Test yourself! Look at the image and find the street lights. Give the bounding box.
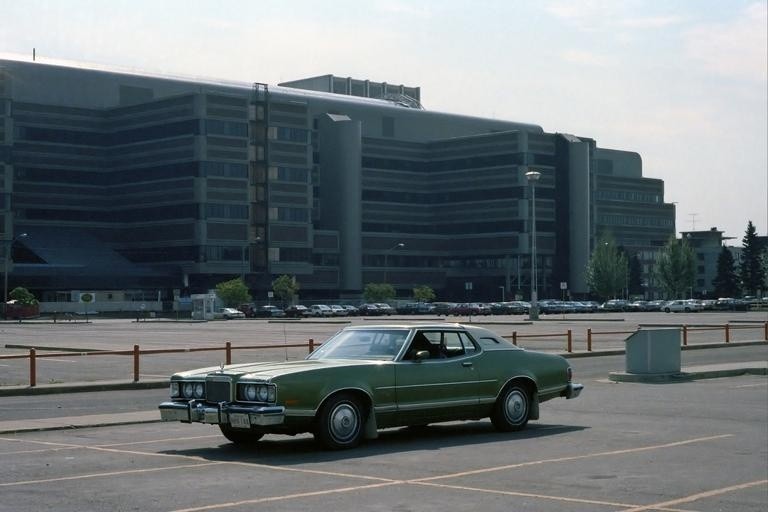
[241,236,262,281]
[522,168,545,320]
[384,243,404,282]
[4,231,29,319]
[498,286,505,302]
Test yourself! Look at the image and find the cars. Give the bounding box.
[156,319,586,444]
